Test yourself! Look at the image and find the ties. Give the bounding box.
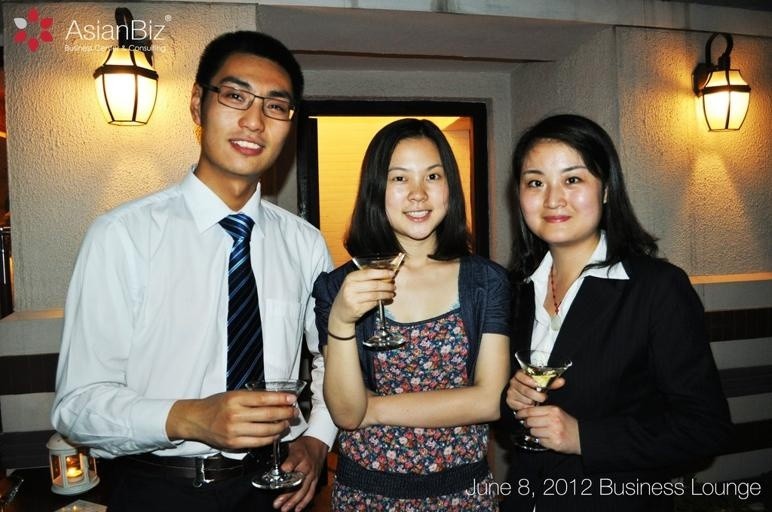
[218,215,269,394]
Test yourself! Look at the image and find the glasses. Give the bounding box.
[204,82,300,122]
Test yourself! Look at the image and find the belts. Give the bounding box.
[128,453,250,485]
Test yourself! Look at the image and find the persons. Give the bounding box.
[52,30,338,511]
[488,114,734,510]
[313,119,512,511]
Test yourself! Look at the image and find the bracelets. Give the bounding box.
[327,326,356,342]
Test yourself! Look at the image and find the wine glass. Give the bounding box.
[352,253,407,348]
[246,378,306,490]
[514,350,573,451]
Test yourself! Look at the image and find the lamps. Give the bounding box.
[690,30,751,133]
[91,7,159,128]
